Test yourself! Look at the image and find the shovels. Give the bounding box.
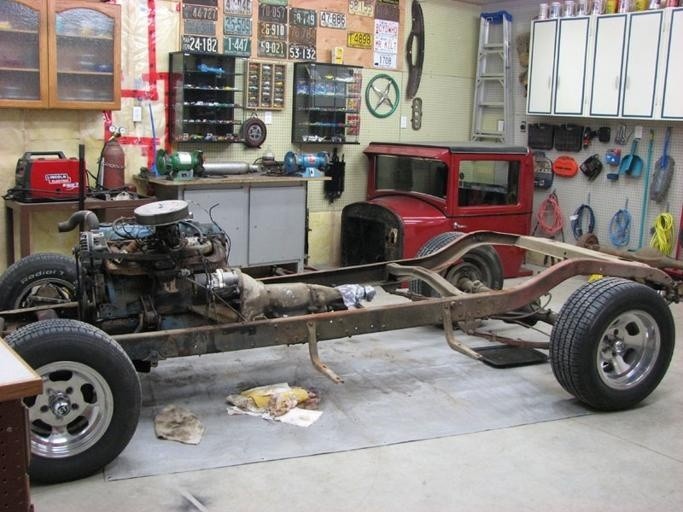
[619,139,643,177]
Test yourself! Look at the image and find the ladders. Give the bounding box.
[470,10,514,145]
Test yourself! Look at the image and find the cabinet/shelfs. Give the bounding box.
[168,50,250,144]
[525,7,682,122]
[292,61,364,144]
[0,0,122,110]
[4,193,157,268]
[243,60,288,111]
[0,333,44,512]
[155,181,308,273]
